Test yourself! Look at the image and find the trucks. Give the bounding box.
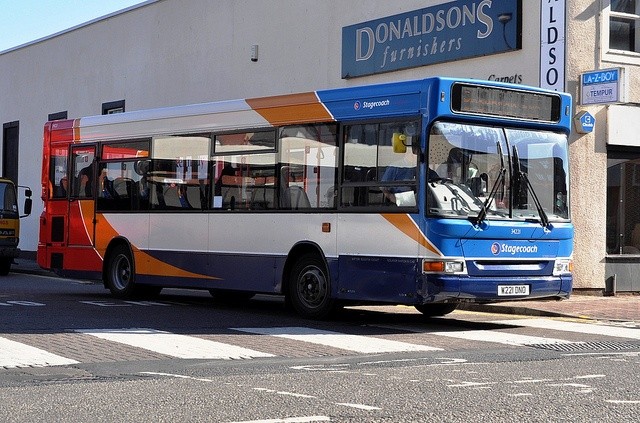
[0,175,34,276]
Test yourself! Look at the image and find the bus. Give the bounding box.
[36,76,576,323]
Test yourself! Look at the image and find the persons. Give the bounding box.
[76,156,100,198]
[434,147,481,197]
[380,141,428,204]
